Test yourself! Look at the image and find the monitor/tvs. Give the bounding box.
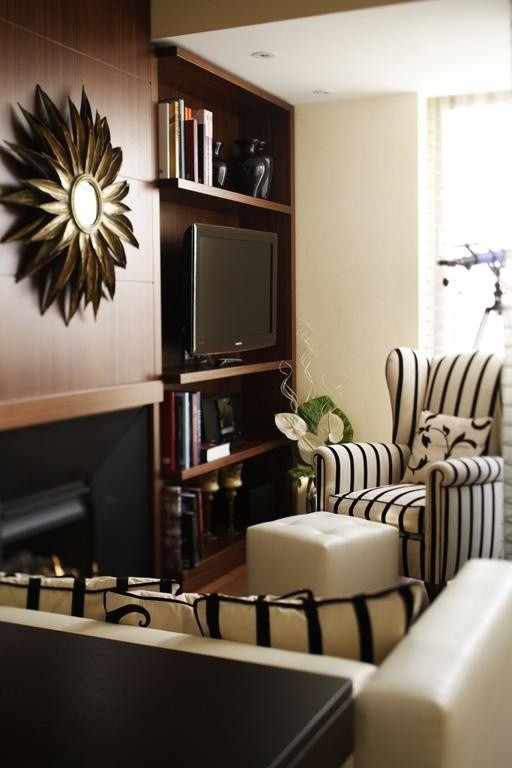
[182,221,278,356]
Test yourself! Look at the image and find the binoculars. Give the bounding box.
[437,245,512,286]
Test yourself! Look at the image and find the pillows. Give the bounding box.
[402,408,494,488]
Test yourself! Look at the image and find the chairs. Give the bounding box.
[308,346,510,597]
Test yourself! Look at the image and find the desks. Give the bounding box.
[0,622,356,767]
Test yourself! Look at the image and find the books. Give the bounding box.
[167,390,243,470]
[160,98,214,188]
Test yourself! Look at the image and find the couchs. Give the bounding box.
[0,556,512,767]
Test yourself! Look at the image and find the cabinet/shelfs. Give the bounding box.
[151,40,300,596]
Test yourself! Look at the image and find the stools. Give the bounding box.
[245,510,399,598]
